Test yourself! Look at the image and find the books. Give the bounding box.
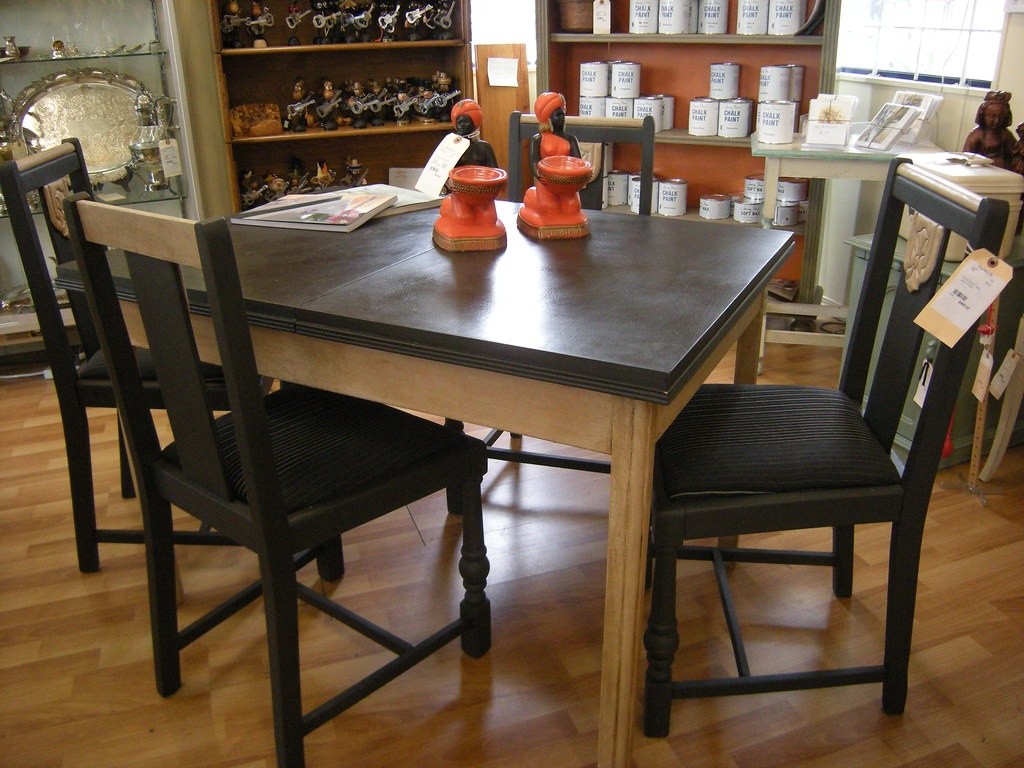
[231,182,445,234]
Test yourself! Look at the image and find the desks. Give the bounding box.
[52,199,794,768]
[751,129,952,376]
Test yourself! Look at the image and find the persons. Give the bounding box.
[220,0,457,49]
[964,89,1024,235]
[281,72,453,131]
[239,157,368,210]
[524,91,594,216]
[438,99,508,227]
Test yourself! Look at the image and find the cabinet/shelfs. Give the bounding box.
[535,1,842,331]
[206,0,470,218]
[0,1,210,362]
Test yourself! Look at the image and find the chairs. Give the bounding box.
[62,201,495,768]
[643,155,1009,740]
[1,137,276,576]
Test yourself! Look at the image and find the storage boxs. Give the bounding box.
[893,151,1024,262]
[846,235,1024,470]
